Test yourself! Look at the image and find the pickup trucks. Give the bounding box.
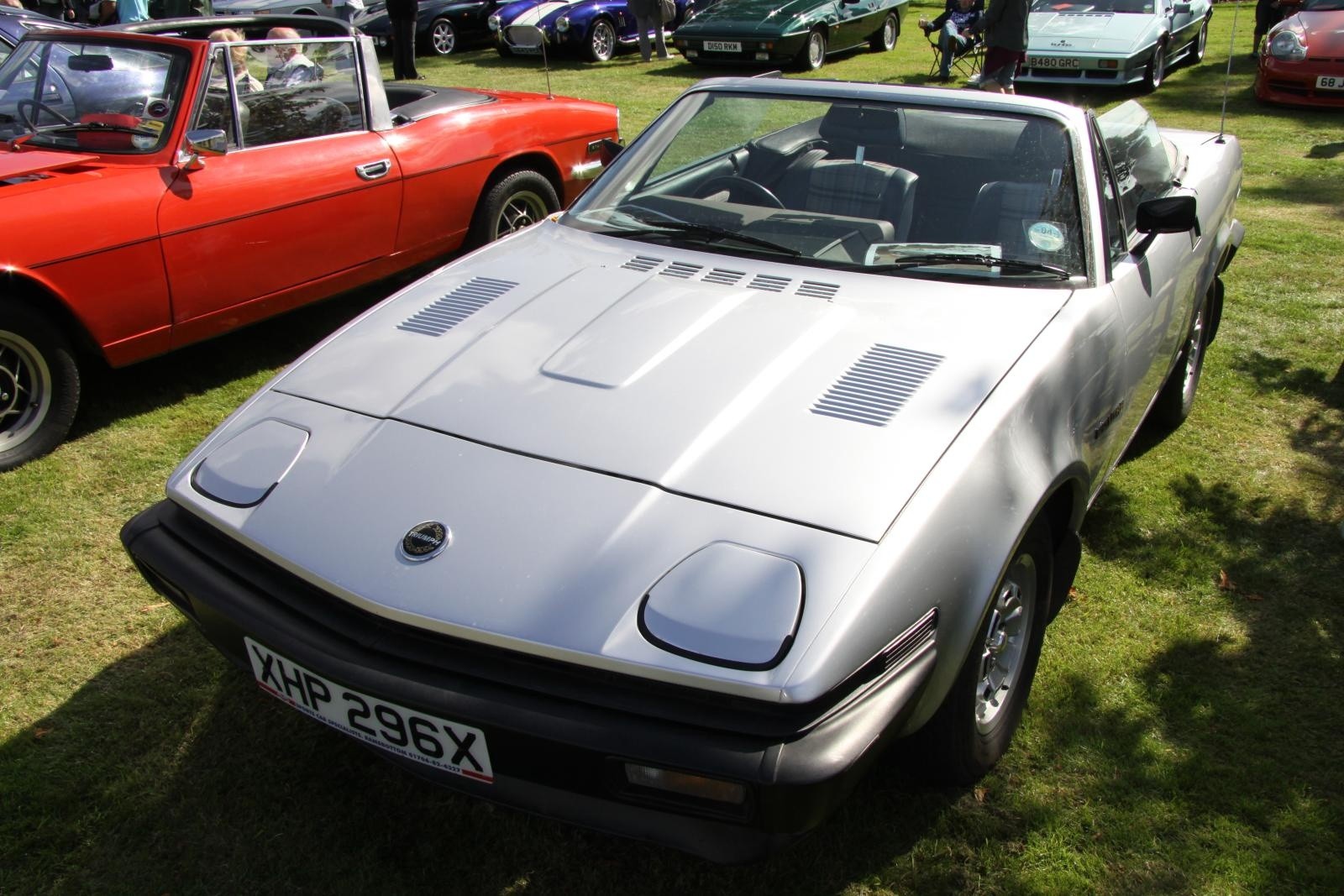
[117,68,1247,868]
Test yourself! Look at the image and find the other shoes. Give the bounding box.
[645,57,651,62]
[939,76,949,84]
[417,74,427,81]
[946,35,958,51]
[659,53,674,60]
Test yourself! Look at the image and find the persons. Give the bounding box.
[626,0,721,62]
[207,28,264,95]
[316,0,336,36]
[1249,0,1288,61]
[0,0,215,27]
[918,0,1034,95]
[385,0,428,81]
[263,27,324,91]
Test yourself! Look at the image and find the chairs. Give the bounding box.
[776,102,920,243]
[924,0,986,79]
[970,120,1085,265]
[124,87,352,148]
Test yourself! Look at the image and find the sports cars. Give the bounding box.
[0,10,628,474]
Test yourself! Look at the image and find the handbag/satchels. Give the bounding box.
[660,0,676,23]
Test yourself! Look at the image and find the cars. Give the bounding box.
[194,0,696,65]
[0,6,172,145]
[1251,0,1344,112]
[669,0,913,72]
[1013,1,1216,95]
